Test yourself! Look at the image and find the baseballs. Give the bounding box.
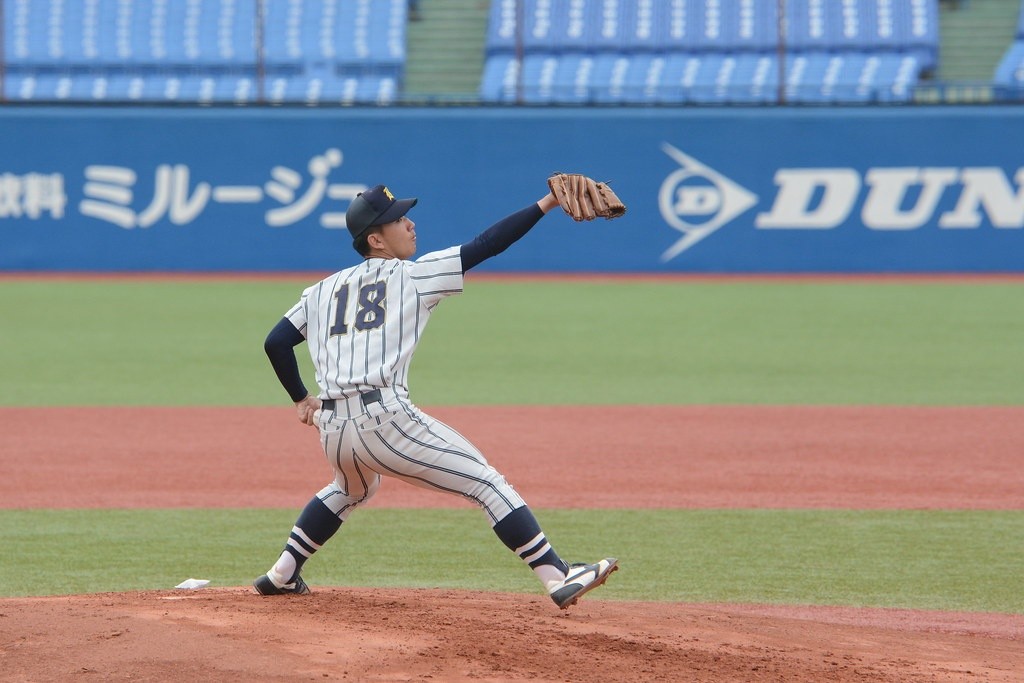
[313,409,320,426]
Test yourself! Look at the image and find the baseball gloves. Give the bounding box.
[547,172,626,222]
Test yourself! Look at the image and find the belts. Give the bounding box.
[322,389,381,410]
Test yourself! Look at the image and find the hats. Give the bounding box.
[346,185,417,240]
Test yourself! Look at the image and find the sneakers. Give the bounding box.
[550,558,619,610]
[253,567,311,596]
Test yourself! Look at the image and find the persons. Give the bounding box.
[253,171,627,610]
